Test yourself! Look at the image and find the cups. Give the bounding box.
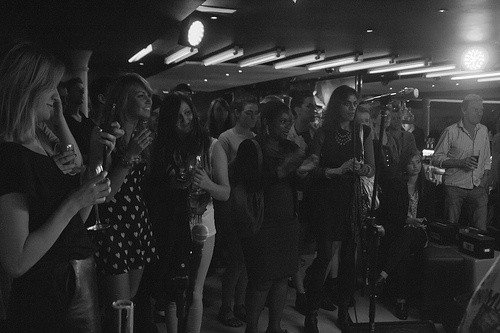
[53,143,75,175]
[470,150,480,169]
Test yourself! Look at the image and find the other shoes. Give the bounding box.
[319,289,337,312]
[233,304,249,322]
[217,311,242,327]
[396,302,408,320]
[349,296,354,308]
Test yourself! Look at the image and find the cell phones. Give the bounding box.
[102,103,118,132]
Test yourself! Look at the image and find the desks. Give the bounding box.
[458,248,500,333]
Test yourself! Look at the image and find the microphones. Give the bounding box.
[360,87,420,104]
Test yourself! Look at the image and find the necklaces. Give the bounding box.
[335,138,352,144]
[336,128,350,138]
[338,130,352,141]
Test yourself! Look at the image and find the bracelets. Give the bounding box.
[120,155,133,169]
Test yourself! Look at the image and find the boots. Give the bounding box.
[304,311,319,333]
[339,305,356,333]
[295,293,318,317]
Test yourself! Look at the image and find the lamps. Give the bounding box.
[126,19,500,82]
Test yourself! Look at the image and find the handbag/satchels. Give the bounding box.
[358,124,380,210]
[231,138,264,224]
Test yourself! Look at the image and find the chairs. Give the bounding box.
[364,225,434,333]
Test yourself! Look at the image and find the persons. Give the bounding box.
[0,40,500,333]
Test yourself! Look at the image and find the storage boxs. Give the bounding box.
[456,229,496,259]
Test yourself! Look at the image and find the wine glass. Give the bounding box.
[80,161,111,230]
[187,154,207,196]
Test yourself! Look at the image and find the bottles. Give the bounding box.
[423,136,437,150]
